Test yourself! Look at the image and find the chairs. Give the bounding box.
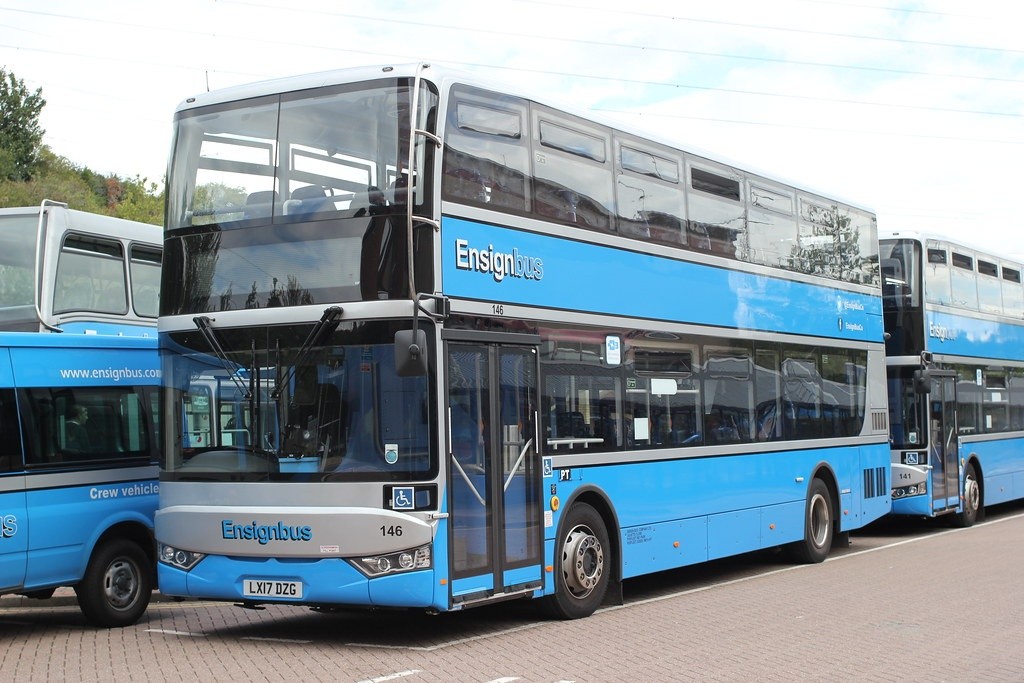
[289,184,337,216]
[135,283,158,316]
[349,187,386,210]
[244,191,281,220]
[386,176,417,205]
[62,274,94,310]
[96,278,125,312]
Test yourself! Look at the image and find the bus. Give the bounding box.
[0,199,279,629]
[156,58,893,623]
[781,230,1024,529]
